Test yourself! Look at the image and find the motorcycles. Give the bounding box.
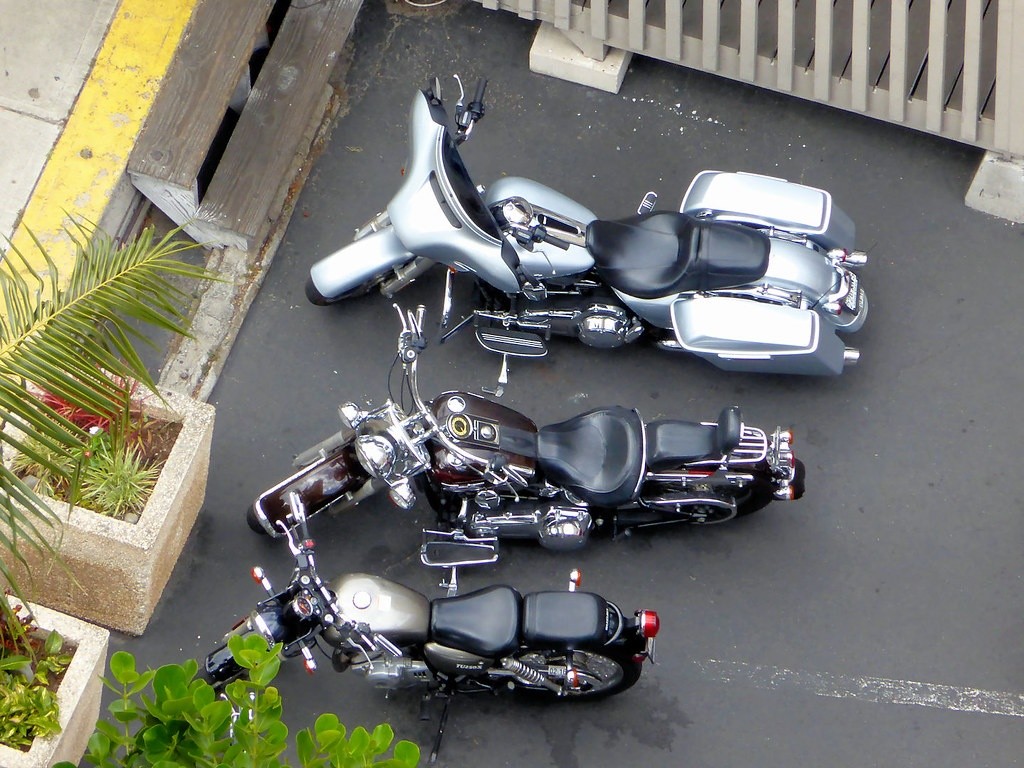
[304,73,869,397]
[242,302,805,596]
[187,491,662,765]
